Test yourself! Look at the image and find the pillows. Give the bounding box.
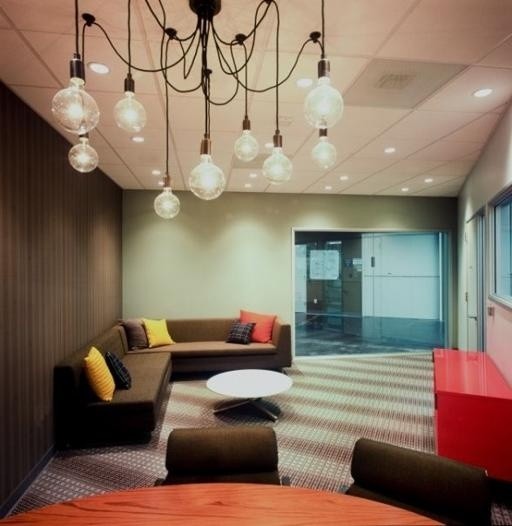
[225,320,255,345]
[105,351,131,389]
[141,318,175,348]
[84,346,116,402]
[239,310,277,343]
[117,318,149,350]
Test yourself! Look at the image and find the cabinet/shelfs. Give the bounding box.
[432,348,512,480]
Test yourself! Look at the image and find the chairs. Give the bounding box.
[154,426,289,486]
[345,438,490,525]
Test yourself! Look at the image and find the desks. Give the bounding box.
[0,482,446,526]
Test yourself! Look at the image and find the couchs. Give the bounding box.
[116,317,291,373]
[52,328,172,432]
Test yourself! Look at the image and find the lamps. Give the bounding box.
[51,0,345,220]
